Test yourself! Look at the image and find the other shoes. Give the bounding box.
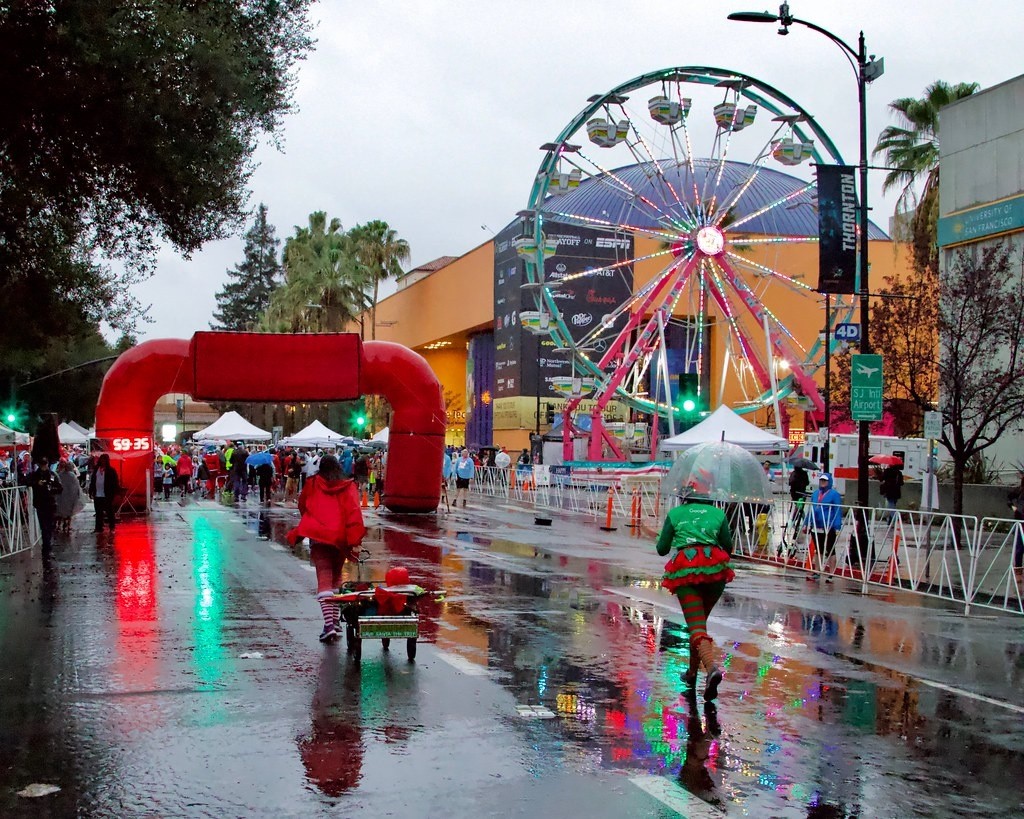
[111,528,115,535]
[260,501,265,503]
[320,623,336,642]
[158,497,162,501]
[680,671,696,689]
[826,577,833,584]
[332,620,344,634]
[164,497,167,501]
[181,491,187,498]
[463,500,466,507]
[241,496,247,501]
[268,500,272,507]
[809,574,821,579]
[154,497,158,501]
[452,501,457,506]
[91,530,104,535]
[703,670,722,702]
[49,550,56,558]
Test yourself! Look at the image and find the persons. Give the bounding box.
[31,457,63,564]
[153,438,529,506]
[714,501,771,537]
[656,467,734,700]
[284,455,366,641]
[874,465,904,528]
[803,473,843,581]
[0,442,95,493]
[88,454,118,533]
[763,460,774,480]
[788,465,809,527]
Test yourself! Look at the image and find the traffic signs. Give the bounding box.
[850,353,888,422]
[7,411,20,428]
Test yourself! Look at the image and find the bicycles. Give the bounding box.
[775,491,815,559]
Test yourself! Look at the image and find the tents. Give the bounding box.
[0,420,31,483]
[67,420,91,435]
[660,403,788,535]
[542,417,592,465]
[56,419,89,454]
[362,426,390,449]
[192,409,273,456]
[279,416,363,446]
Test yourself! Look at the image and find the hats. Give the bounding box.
[819,475,829,481]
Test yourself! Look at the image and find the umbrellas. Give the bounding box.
[660,429,775,508]
[495,452,511,469]
[868,454,904,466]
[778,456,820,470]
[245,452,275,465]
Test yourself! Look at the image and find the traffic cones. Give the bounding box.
[360,490,371,509]
[371,491,381,509]
[521,474,531,490]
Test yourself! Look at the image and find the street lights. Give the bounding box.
[728,2,883,565]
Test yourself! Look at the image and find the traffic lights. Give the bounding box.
[18,402,29,425]
[356,412,368,429]
